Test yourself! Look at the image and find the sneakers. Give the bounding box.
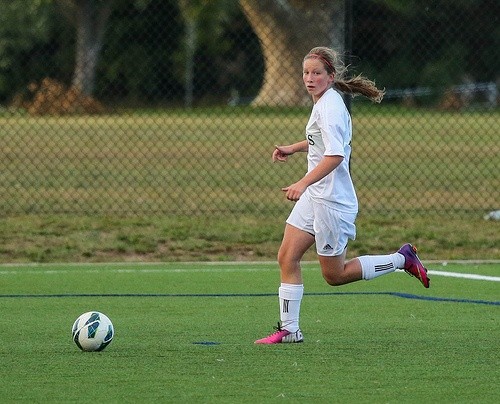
[397,243,431,289]
[254,322,304,344]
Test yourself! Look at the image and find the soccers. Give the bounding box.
[71,311,115,352]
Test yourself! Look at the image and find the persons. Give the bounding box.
[254,46,430,344]
